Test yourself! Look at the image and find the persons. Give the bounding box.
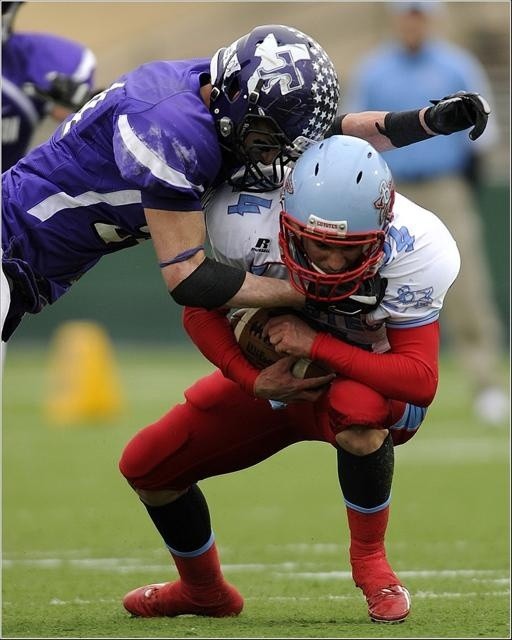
[0,0,100,171]
[1,25,491,354]
[339,4,511,436]
[115,136,462,620]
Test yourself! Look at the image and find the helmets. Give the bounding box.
[207,21,342,197]
[274,133,398,305]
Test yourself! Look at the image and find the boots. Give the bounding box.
[122,528,246,620]
[343,493,413,626]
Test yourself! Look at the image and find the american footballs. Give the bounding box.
[230,308,341,393]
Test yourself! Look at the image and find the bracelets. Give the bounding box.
[383,109,430,148]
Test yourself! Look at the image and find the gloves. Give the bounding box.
[300,267,388,319]
[418,87,491,142]
